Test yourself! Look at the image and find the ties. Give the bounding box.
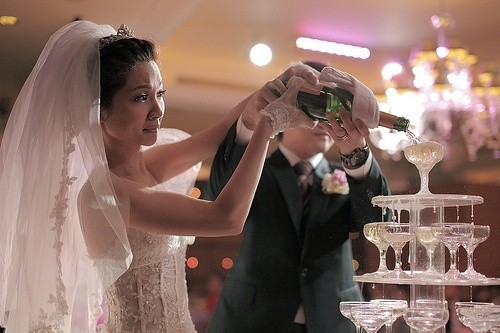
[294,161,315,232]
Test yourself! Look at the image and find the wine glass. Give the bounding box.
[405,142,445,194]
[339,299,500,333]
[363,221,490,279]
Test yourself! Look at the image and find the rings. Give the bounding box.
[338,132,349,142]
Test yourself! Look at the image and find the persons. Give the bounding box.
[187,266,500,333]
[77,24,319,333]
[205,61,391,333]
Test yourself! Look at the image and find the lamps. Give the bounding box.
[368,14,500,167]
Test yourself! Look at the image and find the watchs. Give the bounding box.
[339,141,370,170]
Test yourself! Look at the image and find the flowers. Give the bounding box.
[321,169,351,195]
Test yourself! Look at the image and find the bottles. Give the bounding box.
[281,77,409,134]
